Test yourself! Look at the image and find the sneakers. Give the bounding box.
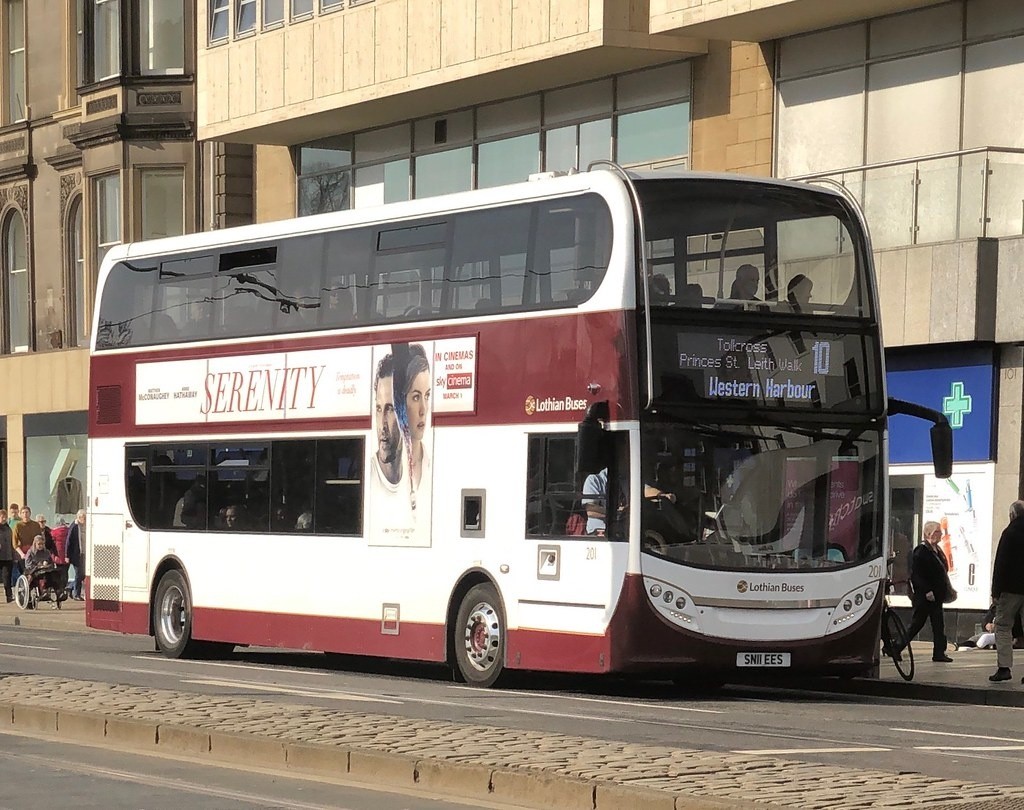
[988,668,1012,681]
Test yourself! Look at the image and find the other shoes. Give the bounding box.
[932,657,953,662]
[57,592,69,603]
[947,641,958,651]
[7,595,15,602]
[66,590,73,599]
[891,643,903,661]
[37,591,49,602]
[74,595,85,601]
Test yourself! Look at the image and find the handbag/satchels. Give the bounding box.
[943,572,957,603]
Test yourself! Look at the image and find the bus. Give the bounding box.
[82,159,954,688]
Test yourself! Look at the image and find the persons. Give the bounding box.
[224,505,245,531]
[788,274,814,314]
[717,264,769,310]
[1,504,86,601]
[988,500,1024,685]
[889,521,958,662]
[373,346,431,498]
[330,281,354,325]
[582,435,676,538]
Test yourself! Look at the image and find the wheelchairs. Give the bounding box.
[15,555,70,610]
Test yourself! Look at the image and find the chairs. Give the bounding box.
[159,313,178,338]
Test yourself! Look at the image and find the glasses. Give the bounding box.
[38,520,46,524]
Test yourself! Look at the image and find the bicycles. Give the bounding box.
[881,551,914,681]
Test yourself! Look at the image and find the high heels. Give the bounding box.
[882,645,891,656]
